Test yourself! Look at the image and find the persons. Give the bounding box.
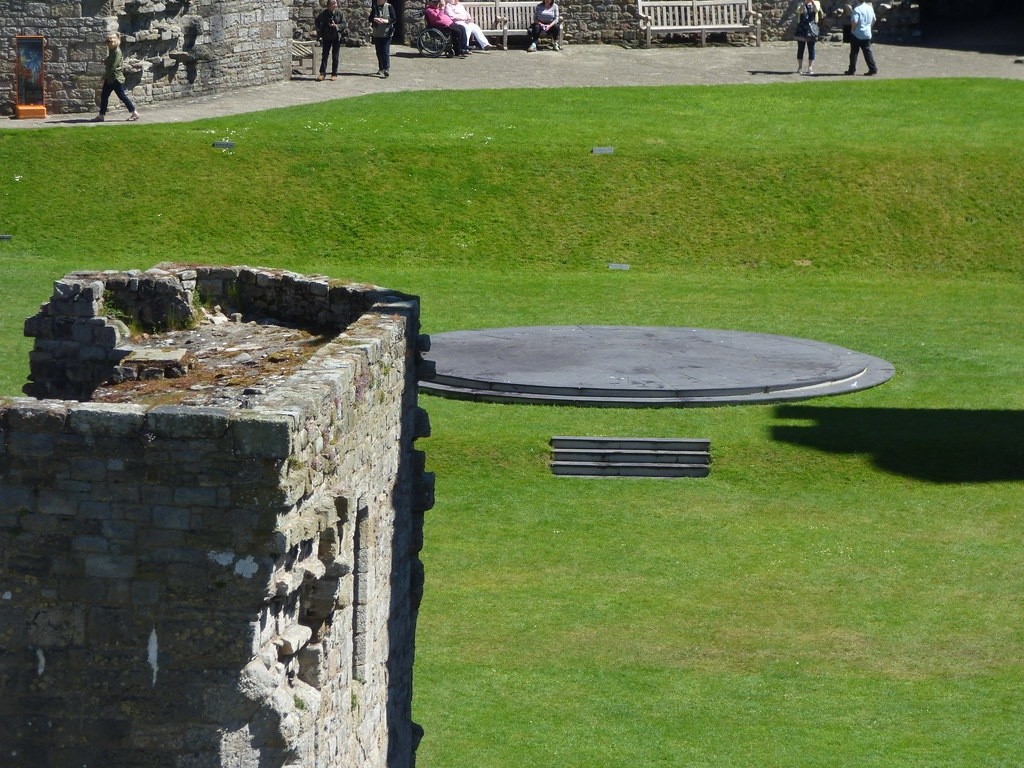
[444,0,497,51]
[91,34,140,122]
[368,0,396,77]
[425,0,476,55]
[794,0,825,74]
[844,0,877,75]
[528,0,560,51]
[315,0,346,81]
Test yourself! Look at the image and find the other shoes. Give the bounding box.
[844,71,856,75]
[552,43,560,51]
[527,46,538,52]
[467,46,475,51]
[126,114,140,121]
[331,75,337,80]
[377,70,383,75]
[864,70,877,76]
[384,70,389,77]
[317,75,325,81]
[91,116,104,122]
[797,70,803,74]
[484,44,497,51]
[808,69,813,74]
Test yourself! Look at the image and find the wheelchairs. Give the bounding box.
[415,8,459,60]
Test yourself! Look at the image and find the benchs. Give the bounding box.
[292,40,321,74]
[461,2,564,51]
[637,0,763,49]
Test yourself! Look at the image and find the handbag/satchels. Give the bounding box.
[805,4,820,38]
